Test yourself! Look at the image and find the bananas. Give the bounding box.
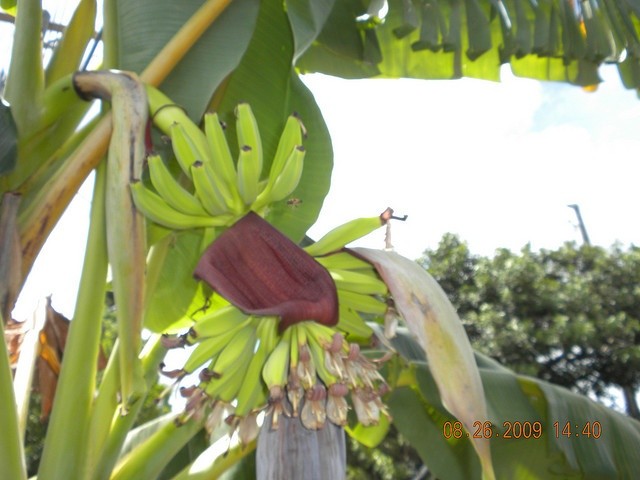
[129,101,304,231]
[182,216,388,419]
